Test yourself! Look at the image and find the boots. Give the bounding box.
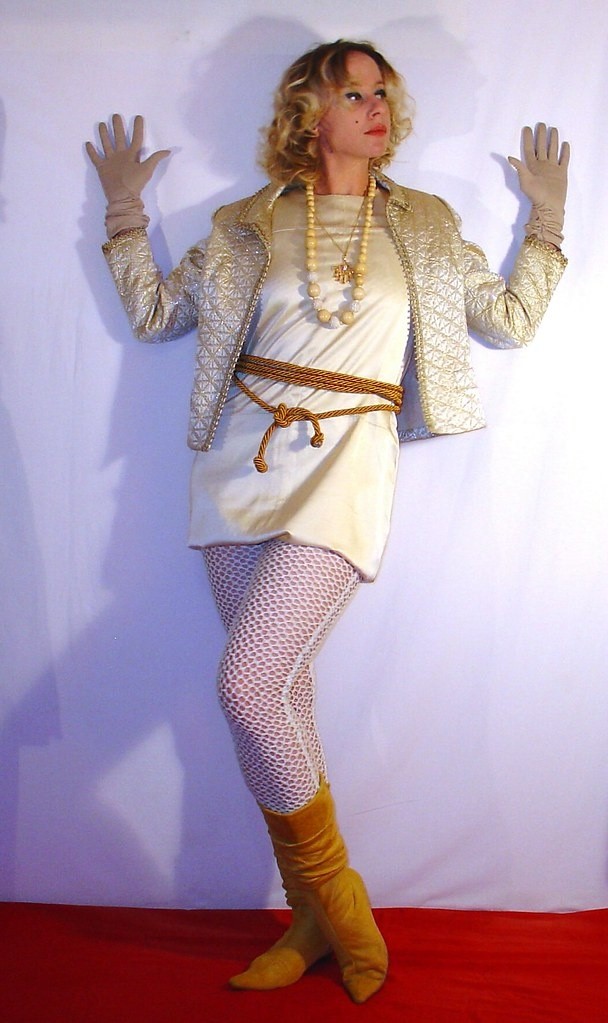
[255,770,390,1005]
[230,828,336,991]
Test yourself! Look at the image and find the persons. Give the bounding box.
[85,40,571,1004]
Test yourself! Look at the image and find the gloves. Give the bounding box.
[86,112,171,241]
[507,122,571,251]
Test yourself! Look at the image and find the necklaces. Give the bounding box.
[301,173,377,329]
